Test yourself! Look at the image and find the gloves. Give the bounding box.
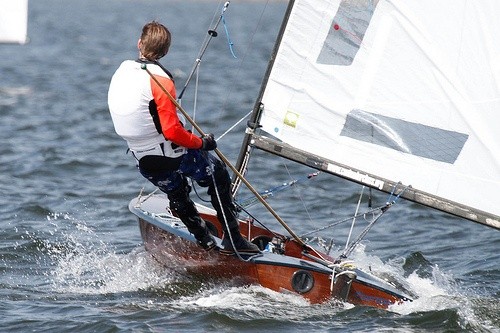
[200,132,218,152]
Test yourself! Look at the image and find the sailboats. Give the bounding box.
[127,0,500,316]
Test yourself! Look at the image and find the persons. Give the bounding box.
[107,20,265,256]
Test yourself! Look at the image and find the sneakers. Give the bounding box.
[195,235,218,258]
[218,227,262,258]
